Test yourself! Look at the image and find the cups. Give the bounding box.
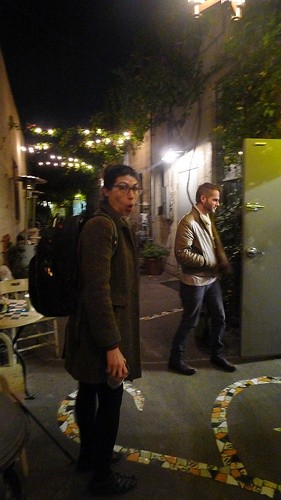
[107,364,130,389]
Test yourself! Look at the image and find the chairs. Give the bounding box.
[0,278,62,370]
[0,332,32,500]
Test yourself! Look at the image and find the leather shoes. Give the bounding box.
[210,358,239,372]
[77,450,139,497]
[168,361,198,375]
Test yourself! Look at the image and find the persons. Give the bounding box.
[167,182,238,374]
[64,163,143,495]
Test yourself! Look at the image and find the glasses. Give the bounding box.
[112,182,144,196]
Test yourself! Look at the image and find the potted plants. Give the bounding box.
[142,243,167,275]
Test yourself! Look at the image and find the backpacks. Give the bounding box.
[27,213,119,318]
